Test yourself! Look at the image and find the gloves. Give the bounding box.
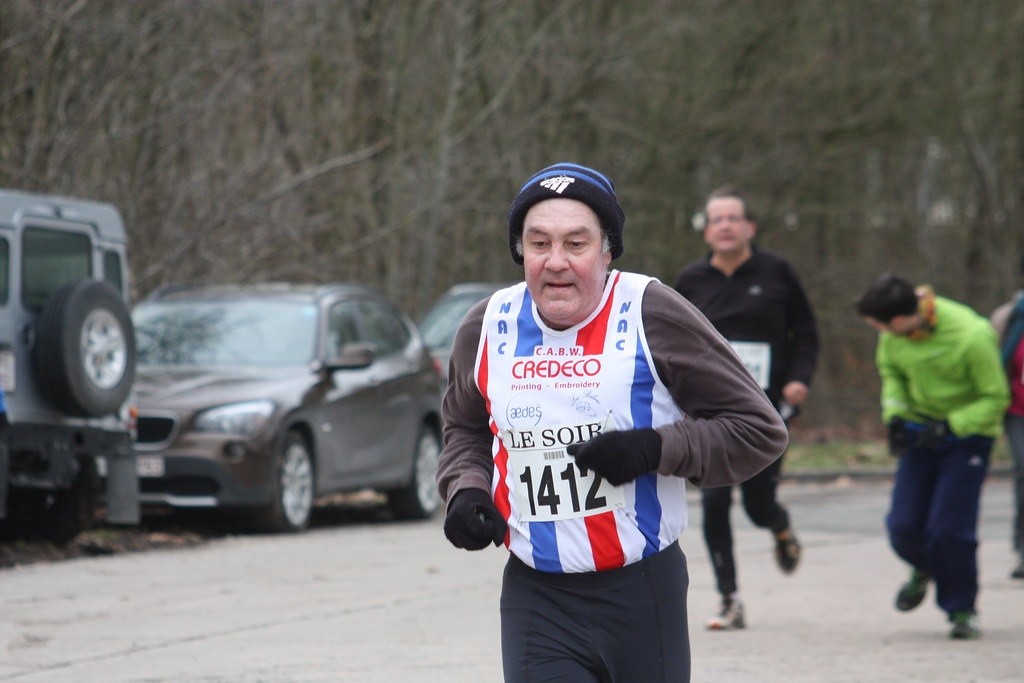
[567,428,662,487]
[444,487,508,553]
[917,422,957,452]
[888,418,909,455]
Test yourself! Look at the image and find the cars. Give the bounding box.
[97,278,445,535]
[419,279,523,383]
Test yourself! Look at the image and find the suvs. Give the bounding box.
[0,186,142,547]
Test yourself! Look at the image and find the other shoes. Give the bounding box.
[774,525,799,572]
[708,597,743,631]
[950,611,974,636]
[896,570,931,612]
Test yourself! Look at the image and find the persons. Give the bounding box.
[854,273,1009,637]
[673,183,818,630]
[989,289,1024,579]
[433,162,788,683]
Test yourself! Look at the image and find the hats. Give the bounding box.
[507,163,625,264]
